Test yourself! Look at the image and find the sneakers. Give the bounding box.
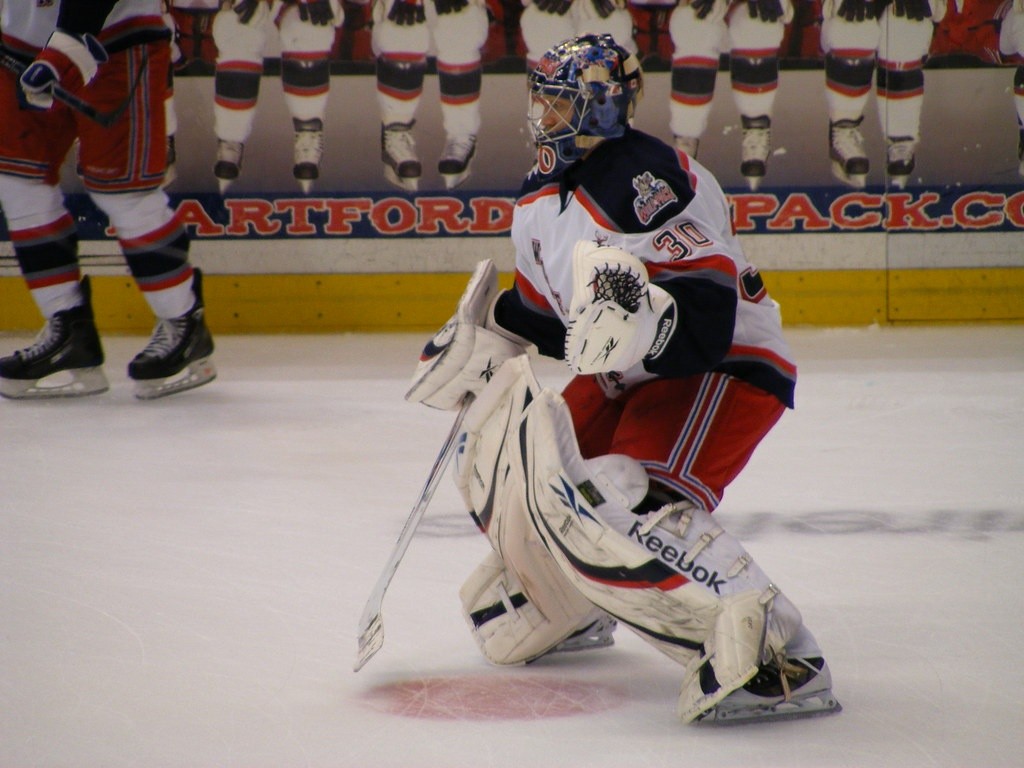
[742,113,772,189]
[290,118,326,193]
[127,266,222,402]
[825,120,873,186]
[887,137,918,187]
[0,273,110,399]
[438,132,481,190]
[211,139,247,192]
[701,632,845,722]
[379,120,426,190]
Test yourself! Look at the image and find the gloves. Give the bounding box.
[385,2,468,28]
[537,1,627,21]
[836,1,933,24]
[691,0,789,25]
[229,1,340,28]
[20,33,107,113]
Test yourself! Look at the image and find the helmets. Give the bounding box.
[529,33,643,139]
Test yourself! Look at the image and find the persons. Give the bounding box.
[0,0,213,379]
[405,33,833,724]
[74,0,1024,180]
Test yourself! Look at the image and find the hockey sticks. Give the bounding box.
[354,393,473,672]
[0,43,153,128]
